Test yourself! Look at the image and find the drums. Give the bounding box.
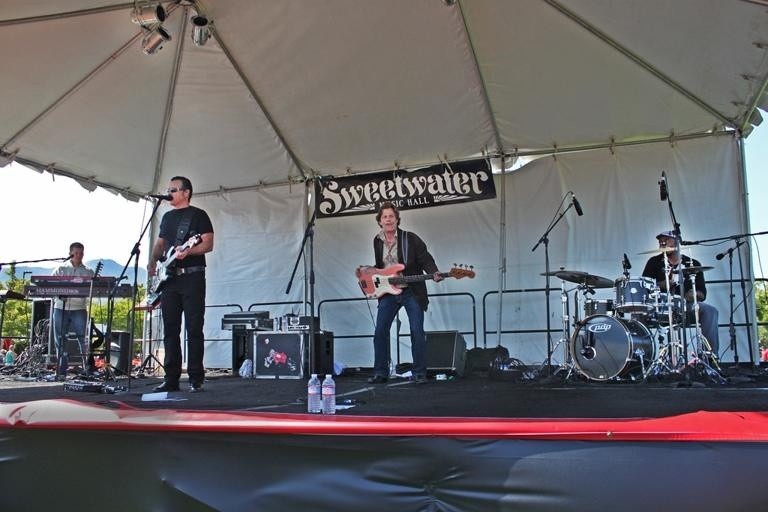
[585,299,615,317]
[615,276,659,316]
[570,314,656,384]
[648,293,688,326]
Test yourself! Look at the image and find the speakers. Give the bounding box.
[424,330,466,377]
[64,255,74,263]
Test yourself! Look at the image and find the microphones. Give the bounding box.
[659,177,667,201]
[716,241,746,261]
[572,192,583,216]
[623,252,631,270]
[150,194,172,201]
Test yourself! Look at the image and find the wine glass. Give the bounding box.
[290,316,300,330]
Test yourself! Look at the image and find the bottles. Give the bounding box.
[322,374,336,414]
[307,374,320,413]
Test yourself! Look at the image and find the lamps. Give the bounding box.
[130,0,216,56]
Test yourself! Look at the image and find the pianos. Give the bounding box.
[22,276,135,298]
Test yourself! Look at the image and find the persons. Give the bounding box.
[354,204,444,384]
[642,231,722,375]
[44,242,95,381]
[147,177,214,393]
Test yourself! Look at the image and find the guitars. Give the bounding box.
[149,234,203,292]
[357,263,476,300]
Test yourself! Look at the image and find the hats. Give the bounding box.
[656,231,676,237]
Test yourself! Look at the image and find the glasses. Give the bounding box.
[165,187,184,193]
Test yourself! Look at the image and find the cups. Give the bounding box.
[282,314,288,331]
[273,315,279,330]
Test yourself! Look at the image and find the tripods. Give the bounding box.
[646,254,725,384]
[538,280,571,378]
[564,294,585,383]
[91,300,136,383]
[133,310,164,375]
[684,274,727,377]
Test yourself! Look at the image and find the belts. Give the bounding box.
[176,265,205,276]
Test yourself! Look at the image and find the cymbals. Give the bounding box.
[539,272,588,277]
[556,271,615,288]
[672,266,714,274]
[637,245,693,256]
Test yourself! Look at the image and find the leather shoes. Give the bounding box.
[153,381,181,393]
[368,374,387,383]
[413,375,427,384]
[188,381,205,394]
